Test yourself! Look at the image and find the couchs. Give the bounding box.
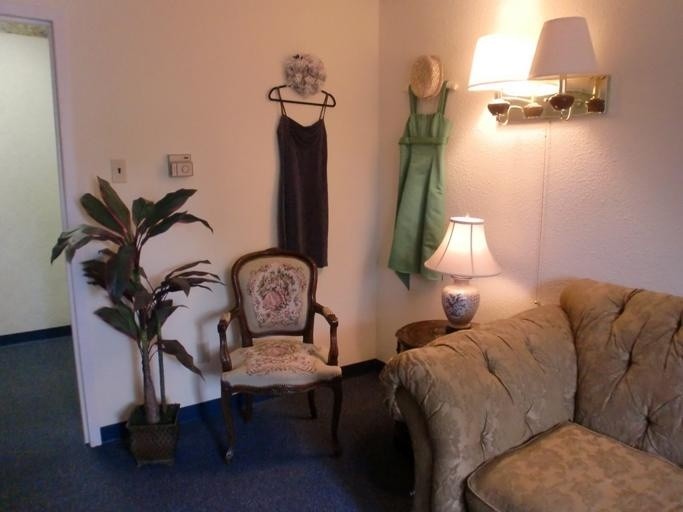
[375,281,683,512]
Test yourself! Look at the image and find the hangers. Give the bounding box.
[268,83,336,108]
[403,78,458,96]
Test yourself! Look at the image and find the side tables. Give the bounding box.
[394,317,482,353]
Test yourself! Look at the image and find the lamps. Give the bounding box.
[466,18,609,124]
[425,215,499,328]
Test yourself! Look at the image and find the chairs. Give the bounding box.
[214,246,344,464]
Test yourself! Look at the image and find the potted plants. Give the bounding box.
[49,175,226,466]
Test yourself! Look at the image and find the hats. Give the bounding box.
[409,56,443,98]
